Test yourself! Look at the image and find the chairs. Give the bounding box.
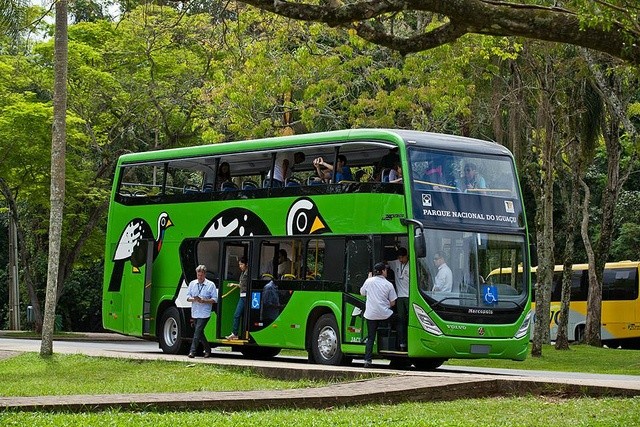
[380,167,392,183]
[118,173,332,197]
[259,269,324,281]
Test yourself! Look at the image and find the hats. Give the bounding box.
[374,262,390,273]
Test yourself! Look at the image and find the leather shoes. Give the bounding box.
[188,352,194,358]
[205,350,211,358]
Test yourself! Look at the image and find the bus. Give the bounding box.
[102,128,538,369]
[484,259,640,349]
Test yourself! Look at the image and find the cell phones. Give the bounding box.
[193,296,201,299]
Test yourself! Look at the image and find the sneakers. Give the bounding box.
[401,347,407,352]
[364,361,372,368]
[225,332,239,340]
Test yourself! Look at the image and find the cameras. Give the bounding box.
[313,158,320,165]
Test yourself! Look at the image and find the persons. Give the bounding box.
[389,162,403,183]
[215,162,232,191]
[458,162,485,193]
[187,265,218,358]
[360,262,399,368]
[423,159,445,191]
[385,247,409,348]
[381,146,403,173]
[225,257,249,340]
[267,152,290,183]
[278,249,291,278]
[432,250,453,292]
[313,154,352,185]
[262,276,280,320]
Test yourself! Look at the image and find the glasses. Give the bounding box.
[464,168,471,172]
[433,258,440,260]
